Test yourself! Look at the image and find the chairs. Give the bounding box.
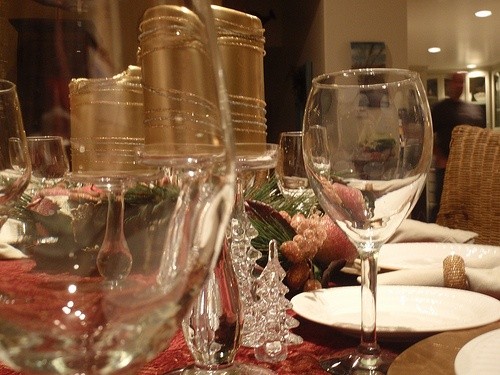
[434,126,499,244]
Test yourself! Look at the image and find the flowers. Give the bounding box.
[1,174,382,293]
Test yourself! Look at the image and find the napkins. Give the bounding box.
[356,218,500,300]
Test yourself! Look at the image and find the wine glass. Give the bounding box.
[302,68,434,375]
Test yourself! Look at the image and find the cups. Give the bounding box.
[275,132,319,211]
[1,0,236,375]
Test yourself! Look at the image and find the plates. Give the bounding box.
[453,328,500,375]
[375,242,500,270]
[357,266,500,298]
[289,284,500,341]
